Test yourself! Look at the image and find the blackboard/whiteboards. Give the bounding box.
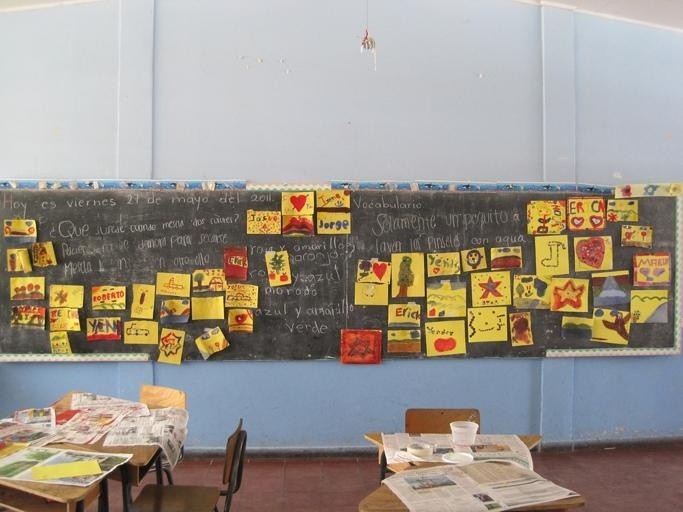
[0,180,683,363]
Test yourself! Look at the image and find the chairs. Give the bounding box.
[406,408,480,435]
[139,386,186,485]
[130,418,247,512]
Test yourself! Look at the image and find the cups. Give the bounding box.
[451,421,479,445]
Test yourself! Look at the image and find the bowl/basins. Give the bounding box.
[410,443,433,456]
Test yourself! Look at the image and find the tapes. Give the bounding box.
[407,441,437,457]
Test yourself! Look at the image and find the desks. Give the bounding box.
[359,433,585,512]
[0,441,113,512]
[40,391,173,512]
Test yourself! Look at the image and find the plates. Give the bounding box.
[444,452,473,462]
